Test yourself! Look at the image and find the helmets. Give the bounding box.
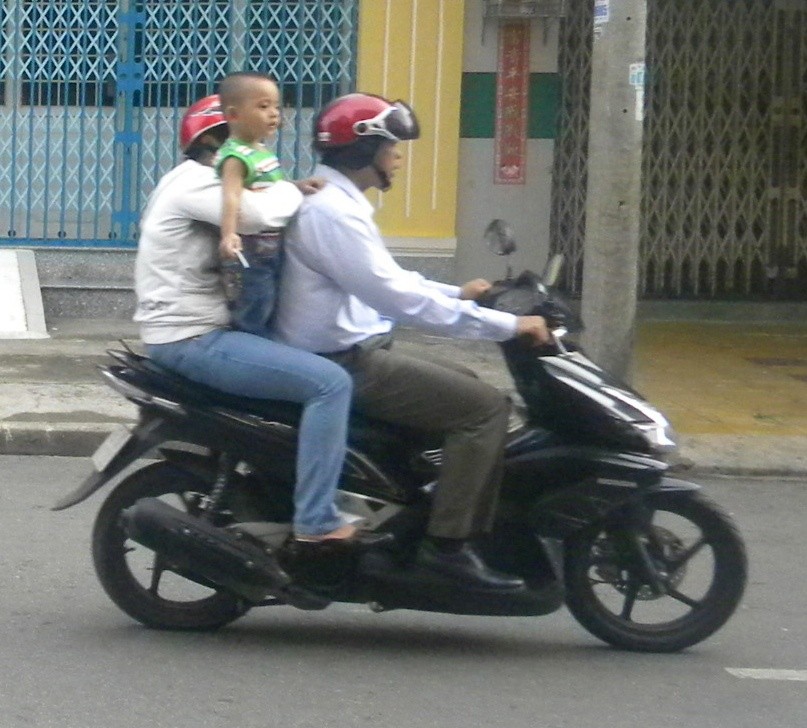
[312,91,420,150]
[179,94,227,154]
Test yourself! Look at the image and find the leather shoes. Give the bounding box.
[415,539,527,593]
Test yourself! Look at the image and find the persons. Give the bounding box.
[273,92,547,597]
[213,71,327,338]
[131,92,371,543]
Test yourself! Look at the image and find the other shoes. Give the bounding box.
[293,527,395,552]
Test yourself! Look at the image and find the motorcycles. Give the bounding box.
[51,216,751,653]
